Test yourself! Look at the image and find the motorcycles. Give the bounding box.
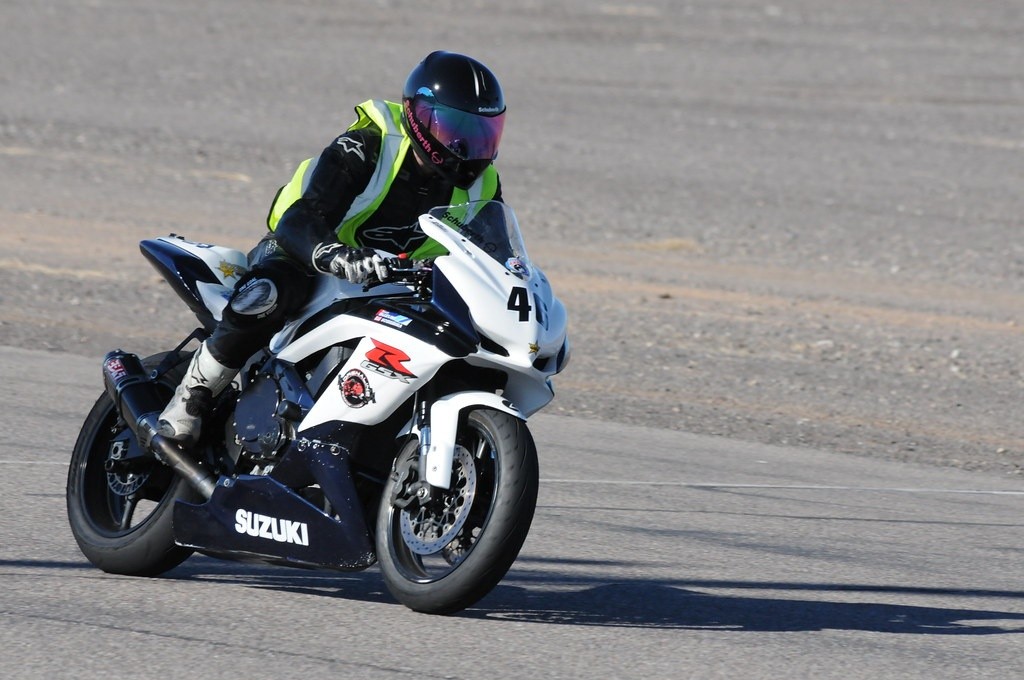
[67,200,571,615]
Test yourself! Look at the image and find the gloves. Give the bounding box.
[312,242,403,284]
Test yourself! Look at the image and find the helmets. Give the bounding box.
[399,50,508,191]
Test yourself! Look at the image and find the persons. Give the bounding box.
[154,51,519,445]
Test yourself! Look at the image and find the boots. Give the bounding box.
[156,340,242,443]
